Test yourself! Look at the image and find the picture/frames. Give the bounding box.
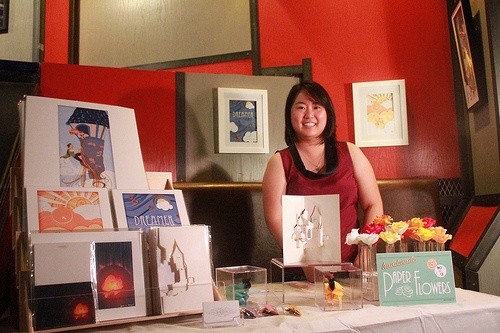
[352,79,408,147]
[217,87,270,153]
[448,0,488,113]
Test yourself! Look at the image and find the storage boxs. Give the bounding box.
[216,265,268,309]
[314,265,363,312]
[270,258,353,306]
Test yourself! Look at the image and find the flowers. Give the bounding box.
[344,214,453,249]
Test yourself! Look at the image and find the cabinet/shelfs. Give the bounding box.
[9,167,222,333]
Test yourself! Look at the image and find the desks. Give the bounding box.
[56,281,500,333]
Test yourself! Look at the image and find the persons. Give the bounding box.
[262,81,383,283]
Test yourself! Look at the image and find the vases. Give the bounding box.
[360,241,445,273]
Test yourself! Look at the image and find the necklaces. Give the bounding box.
[297,143,325,171]
